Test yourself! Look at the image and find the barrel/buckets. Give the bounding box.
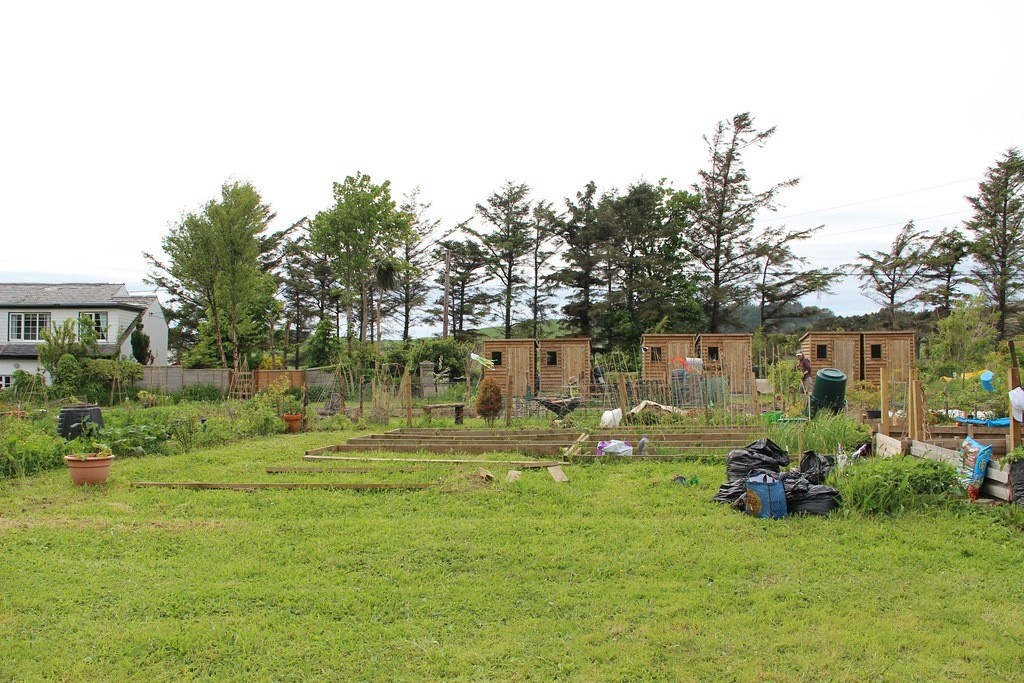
[805,368,847,418]
[980,371,999,390]
[761,411,782,424]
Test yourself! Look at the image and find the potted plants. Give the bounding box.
[283,393,303,433]
[63,412,115,487]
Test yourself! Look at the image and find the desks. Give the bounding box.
[425,404,465,425]
